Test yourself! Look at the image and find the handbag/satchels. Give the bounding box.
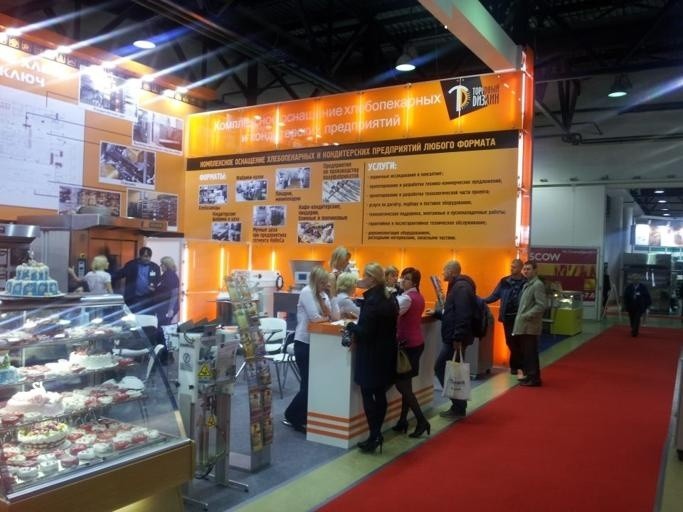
[441,361,472,401]
[396,351,412,374]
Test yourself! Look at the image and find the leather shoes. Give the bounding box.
[440,408,466,418]
[511,368,542,386]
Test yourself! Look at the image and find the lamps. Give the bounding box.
[607,74,630,98]
[394,40,417,73]
[132,14,157,50]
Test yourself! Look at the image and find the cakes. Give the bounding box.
[5,248,60,295]
[0,315,159,479]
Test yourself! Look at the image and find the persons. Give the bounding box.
[68,255,114,294]
[510,261,546,387]
[113,246,159,361]
[601,262,611,315]
[621,273,650,336]
[480,259,527,374]
[150,256,180,365]
[284,246,429,451]
[425,261,476,417]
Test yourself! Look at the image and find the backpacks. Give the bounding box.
[452,278,489,338]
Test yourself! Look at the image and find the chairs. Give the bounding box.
[107,342,164,422]
[233,317,300,398]
[105,313,161,385]
[542,306,557,339]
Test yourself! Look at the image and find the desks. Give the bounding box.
[224,326,282,380]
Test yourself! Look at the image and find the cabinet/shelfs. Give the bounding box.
[550,308,582,336]
[0,304,166,494]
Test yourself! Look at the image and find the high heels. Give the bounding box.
[392,420,408,434]
[408,422,430,438]
[357,433,383,454]
